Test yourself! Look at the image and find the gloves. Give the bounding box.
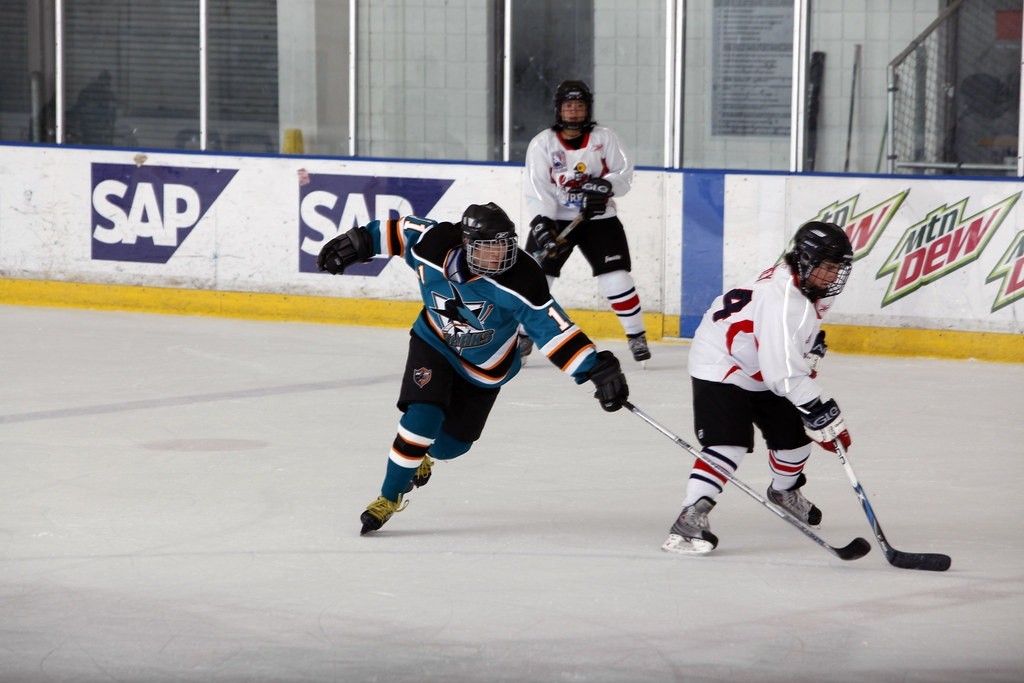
[802,398,851,455]
[530,216,569,259]
[317,226,374,274]
[805,330,828,380]
[567,178,614,222]
[581,351,628,412]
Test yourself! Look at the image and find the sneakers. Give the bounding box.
[412,455,433,487]
[361,493,410,535]
[626,331,652,370]
[767,473,823,531]
[661,496,718,555]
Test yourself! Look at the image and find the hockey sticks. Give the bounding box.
[533,212,585,263]
[624,401,872,562]
[832,437,952,572]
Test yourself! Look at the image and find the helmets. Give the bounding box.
[784,220,854,304]
[462,202,520,277]
[553,79,594,131]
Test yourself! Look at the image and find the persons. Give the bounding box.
[70,70,126,144]
[316,202,629,536]
[661,221,854,557]
[517,80,653,370]
